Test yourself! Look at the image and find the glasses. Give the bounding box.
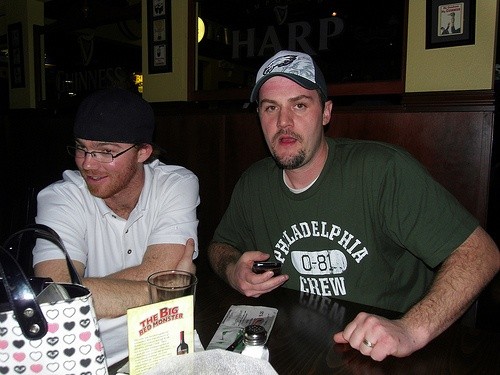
[66,143,141,163]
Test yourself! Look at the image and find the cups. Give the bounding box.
[148,270,198,304]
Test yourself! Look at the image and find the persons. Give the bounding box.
[32,89,201,320]
[207,50,500,361]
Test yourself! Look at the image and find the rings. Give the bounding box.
[363,338,375,348]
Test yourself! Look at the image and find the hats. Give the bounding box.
[72,86,155,144]
[250,50,328,105]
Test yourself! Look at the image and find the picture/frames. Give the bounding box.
[8,21,25,89]
[147,0,172,74]
[426,0,476,49]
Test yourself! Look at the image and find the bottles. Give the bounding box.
[241,325,270,362]
[177,331,189,355]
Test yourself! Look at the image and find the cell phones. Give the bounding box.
[253,262,281,277]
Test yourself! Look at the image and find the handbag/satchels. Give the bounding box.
[0,224,109,375]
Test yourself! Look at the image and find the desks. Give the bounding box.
[196,278,499,374]
